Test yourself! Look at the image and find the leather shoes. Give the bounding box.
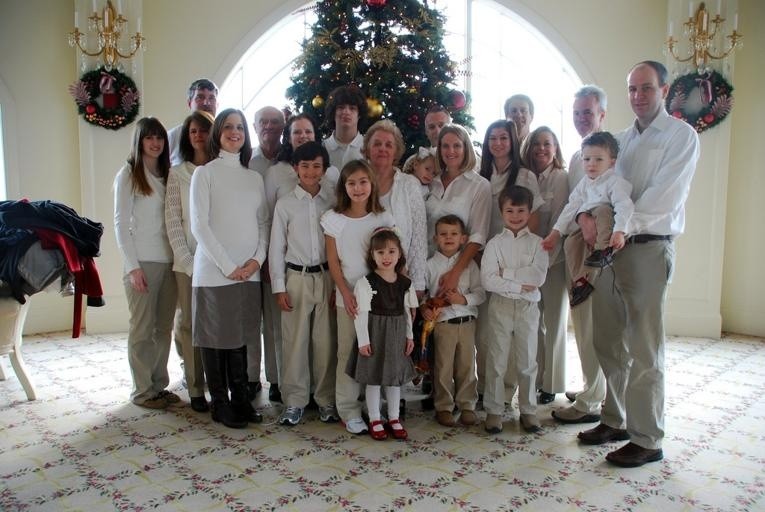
[604,442,668,472]
[138,396,163,414]
[245,378,264,403]
[363,417,384,442]
[386,417,404,439]
[158,384,180,409]
[266,381,281,403]
[570,424,633,447]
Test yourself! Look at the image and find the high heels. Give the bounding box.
[538,389,553,407]
[188,394,206,414]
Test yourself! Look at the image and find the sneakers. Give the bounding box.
[519,410,543,435]
[433,408,456,430]
[458,405,479,430]
[563,387,580,399]
[582,242,624,294]
[275,404,302,427]
[315,403,344,426]
[343,411,370,436]
[563,274,597,310]
[550,405,601,427]
[481,411,504,435]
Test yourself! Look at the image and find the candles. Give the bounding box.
[669,20,674,37]
[689,0,694,18]
[74,9,79,29]
[717,0,721,16]
[104,9,108,27]
[702,12,708,30]
[734,11,738,32]
[137,16,142,33]
[93,0,96,13]
[118,0,122,16]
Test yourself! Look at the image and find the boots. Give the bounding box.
[228,381,267,426]
[208,386,251,431]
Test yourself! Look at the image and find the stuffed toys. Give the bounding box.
[420,304,440,354]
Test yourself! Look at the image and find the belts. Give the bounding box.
[624,229,674,245]
[439,313,475,325]
[284,260,336,274]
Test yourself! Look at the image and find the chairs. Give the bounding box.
[0,293,37,403]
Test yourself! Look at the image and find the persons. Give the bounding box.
[245,104,288,405]
[111,115,180,409]
[419,123,493,416]
[418,105,456,155]
[320,84,370,171]
[503,92,533,172]
[164,108,217,413]
[361,118,430,305]
[477,117,545,418]
[342,224,418,442]
[539,130,634,308]
[549,84,615,424]
[262,113,342,219]
[522,126,581,406]
[423,213,487,429]
[318,159,404,435]
[574,60,700,468]
[188,108,272,429]
[167,78,218,166]
[265,139,339,427]
[401,145,438,201]
